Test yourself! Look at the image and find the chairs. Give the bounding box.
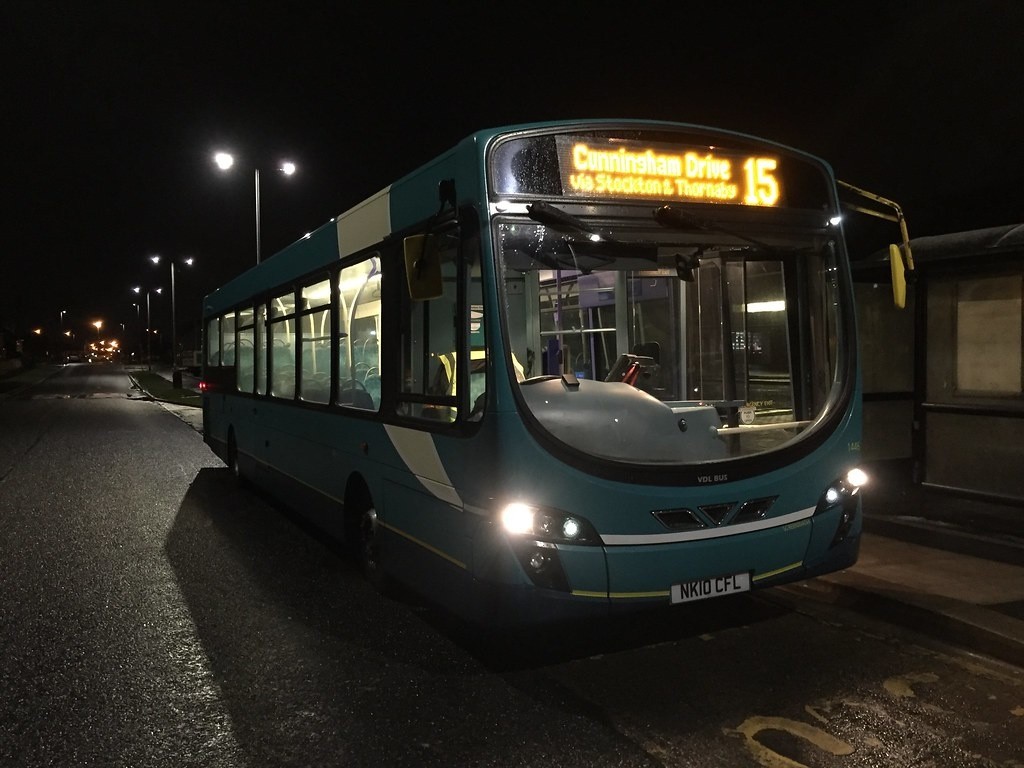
[213,336,380,410]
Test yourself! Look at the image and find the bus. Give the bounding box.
[199,117,915,634]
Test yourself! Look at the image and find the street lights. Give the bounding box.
[210,148,298,265]
[151,254,194,373]
[134,288,163,371]
